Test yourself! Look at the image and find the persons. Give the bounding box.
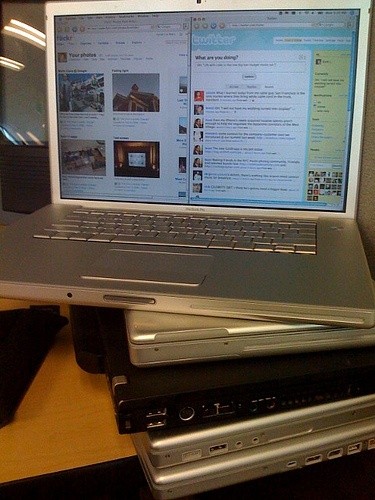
[194,119,201,128]
[193,158,200,167]
[193,183,200,193]
[193,131,201,142]
[193,145,200,155]
[194,171,201,180]
[60,73,72,111]
[195,91,203,101]
[194,105,203,115]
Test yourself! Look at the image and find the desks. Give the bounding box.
[0,297,137,487]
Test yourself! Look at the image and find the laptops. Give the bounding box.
[1,4,375,328]
[138,393,374,468]
[130,433,374,500]
[68,305,374,376]
[104,367,375,436]
[122,307,374,367]
